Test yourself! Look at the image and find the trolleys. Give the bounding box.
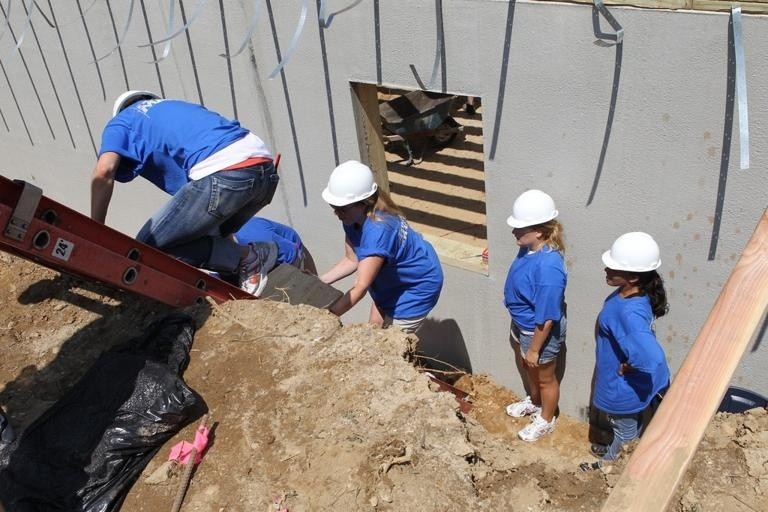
[378,90,466,146]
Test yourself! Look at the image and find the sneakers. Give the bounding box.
[518,410,557,441]
[578,444,611,474]
[505,395,543,418]
[238,240,279,298]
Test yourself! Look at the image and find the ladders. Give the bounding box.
[0,175,473,414]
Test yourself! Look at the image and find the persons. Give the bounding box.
[504,188,567,442]
[233,216,318,276]
[579,231,671,473]
[319,160,443,334]
[91,90,280,299]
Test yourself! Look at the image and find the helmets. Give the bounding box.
[321,160,378,207]
[112,90,162,118]
[505,190,559,228]
[601,232,662,272]
